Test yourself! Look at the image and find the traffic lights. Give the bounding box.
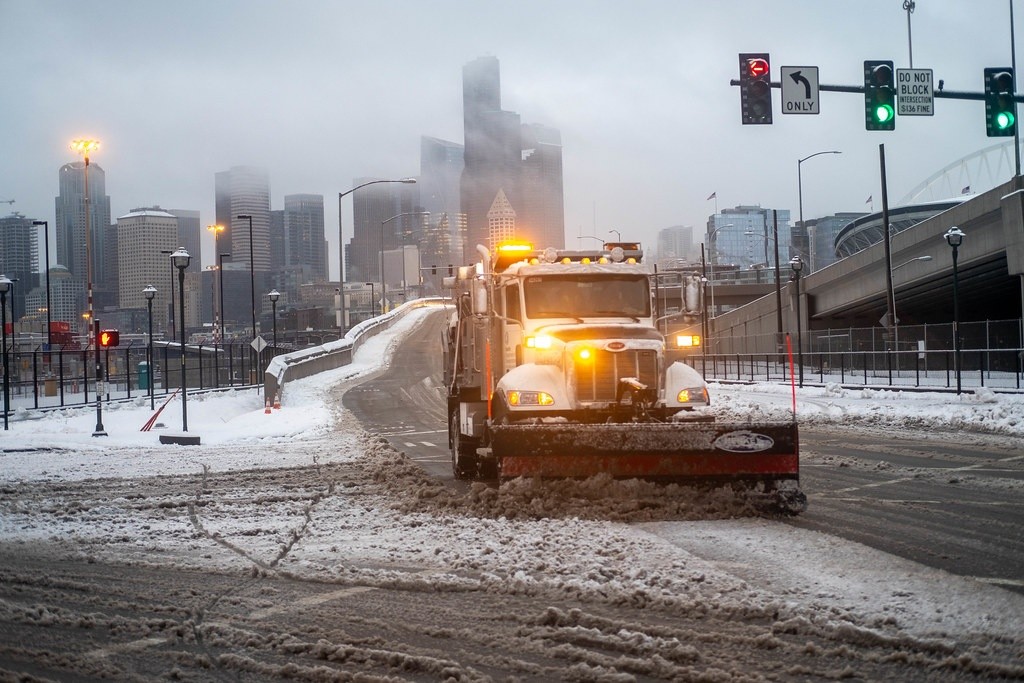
[864,60,895,131]
[984,67,1016,137]
[100,330,119,346]
[739,53,773,125]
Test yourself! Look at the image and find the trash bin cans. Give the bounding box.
[138,361,151,389]
[249,370,257,384]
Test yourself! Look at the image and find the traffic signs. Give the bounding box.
[781,66,821,114]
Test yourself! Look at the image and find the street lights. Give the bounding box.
[237,215,280,381]
[891,255,933,370]
[141,284,158,410]
[663,259,683,335]
[69,139,99,356]
[33,221,51,370]
[789,256,805,387]
[338,178,430,339]
[709,224,733,318]
[162,247,195,431]
[944,227,966,394]
[0,274,20,431]
[207,225,230,356]
[798,151,842,258]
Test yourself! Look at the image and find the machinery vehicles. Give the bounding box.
[441,242,808,518]
